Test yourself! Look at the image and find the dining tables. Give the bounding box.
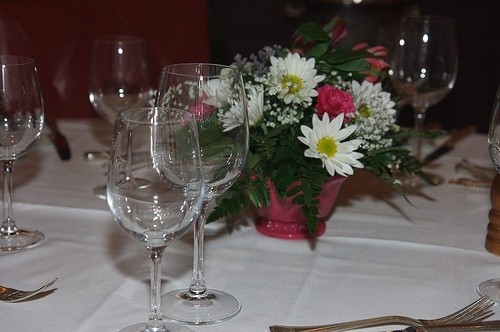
[0,119,500,332]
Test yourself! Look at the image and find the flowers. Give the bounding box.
[171,22,447,236]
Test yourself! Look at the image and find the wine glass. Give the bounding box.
[0,56,46,254]
[148,63,250,325]
[87,35,166,198]
[108,106,204,332]
[475,106,500,302]
[383,9,459,187]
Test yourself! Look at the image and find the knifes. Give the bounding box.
[422,124,478,165]
[45,112,72,161]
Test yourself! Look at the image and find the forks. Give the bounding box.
[269,296,495,332]
[0,276,59,302]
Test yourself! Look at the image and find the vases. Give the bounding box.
[231,166,347,240]
